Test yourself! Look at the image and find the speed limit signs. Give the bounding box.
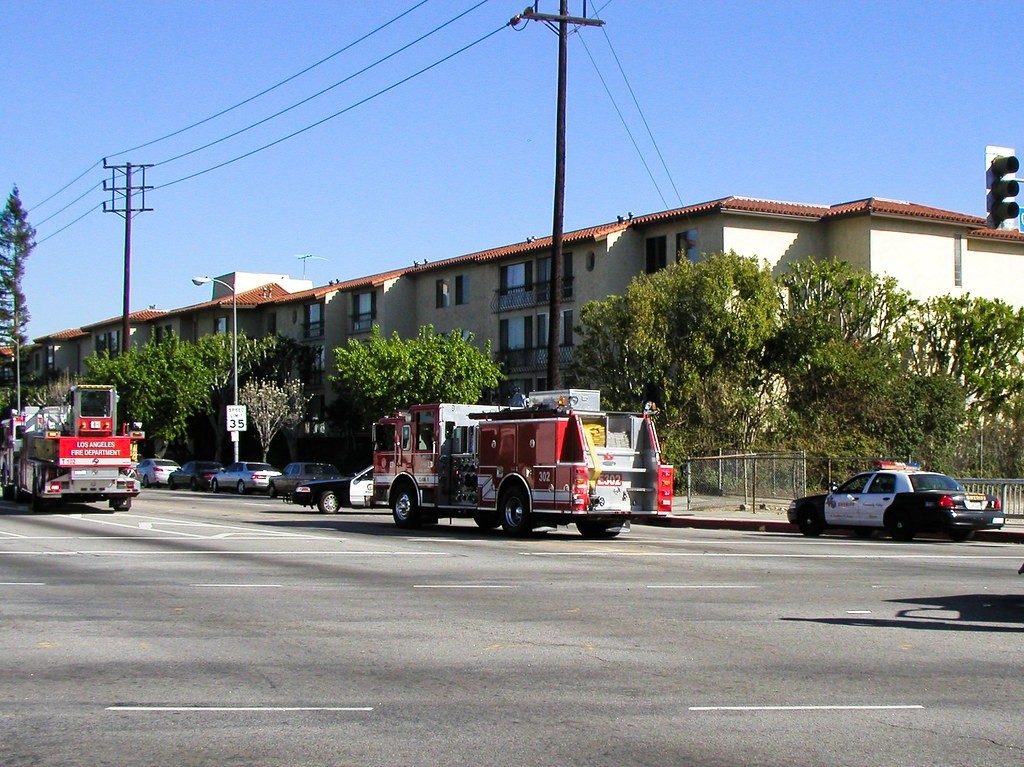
[226,404,247,431]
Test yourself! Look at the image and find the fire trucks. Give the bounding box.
[363,389,675,539]
[0,384,146,515]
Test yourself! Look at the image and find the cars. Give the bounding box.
[292,464,374,515]
[787,460,1006,543]
[267,463,349,499]
[135,458,181,488]
[168,461,227,492]
[210,462,283,495]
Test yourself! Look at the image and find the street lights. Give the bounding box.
[191,275,239,464]
[1,336,21,415]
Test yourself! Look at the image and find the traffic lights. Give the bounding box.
[985,145,1020,231]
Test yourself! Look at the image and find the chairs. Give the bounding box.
[870,482,896,493]
